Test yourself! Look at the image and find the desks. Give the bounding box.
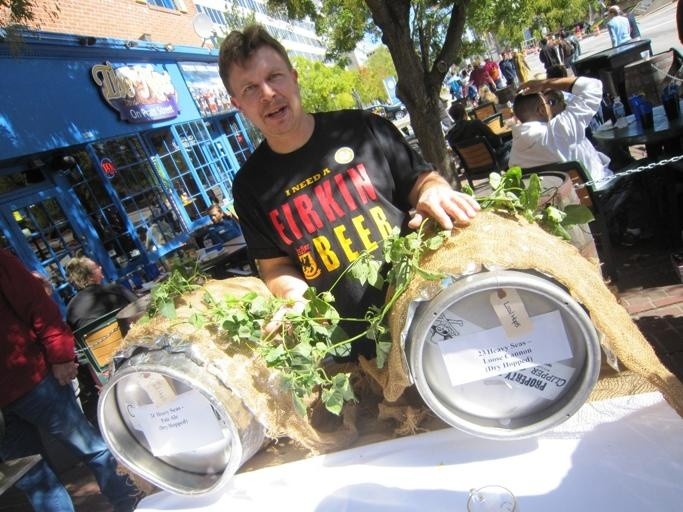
[592,99,682,248]
[133,274,682,511]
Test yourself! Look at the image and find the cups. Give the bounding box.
[639,50,650,60]
[466,482,515,511]
[662,83,678,122]
[639,102,653,129]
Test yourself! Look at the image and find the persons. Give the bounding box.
[444,3,644,112]
[0,245,148,511]
[510,75,658,251]
[543,87,600,152]
[143,208,174,253]
[204,203,241,246]
[443,107,511,176]
[213,20,483,366]
[64,256,140,334]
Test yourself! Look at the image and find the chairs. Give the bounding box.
[67,214,260,387]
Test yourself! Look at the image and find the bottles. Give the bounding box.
[628,92,647,126]
[605,92,628,128]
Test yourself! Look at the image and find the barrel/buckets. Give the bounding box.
[622,51,679,107]
[405,270,604,445]
[473,171,604,279]
[114,277,204,339]
[96,347,273,500]
